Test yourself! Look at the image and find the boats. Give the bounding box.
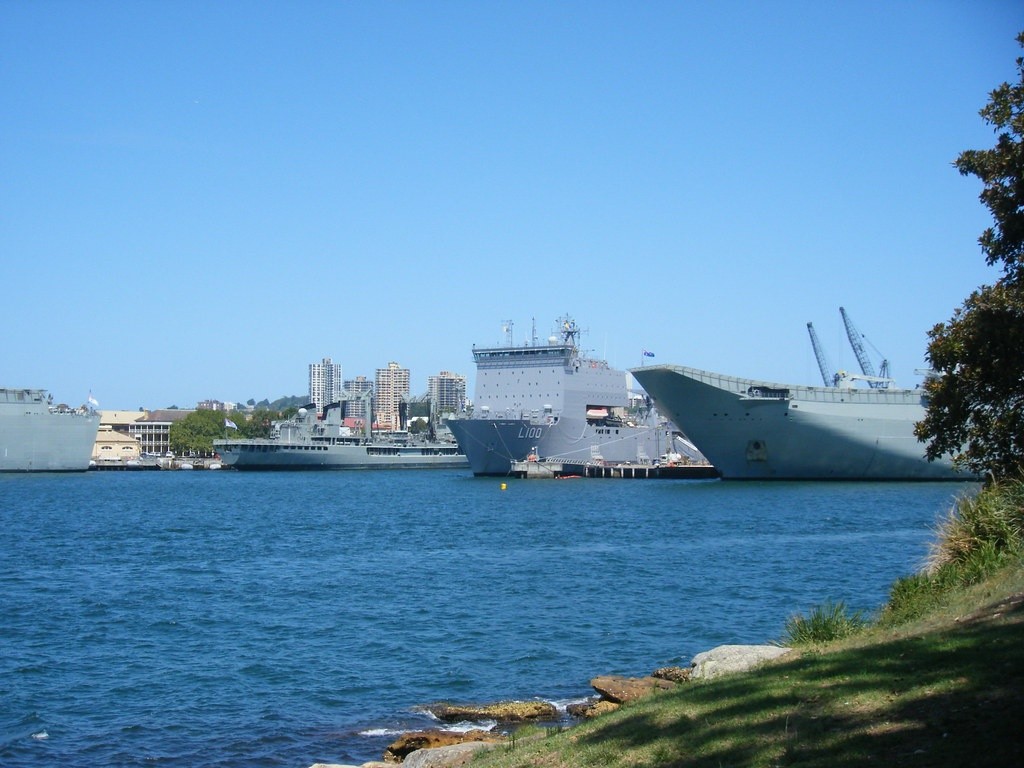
[586,408,608,420]
[606,416,623,425]
[0,387,103,473]
[444,310,715,479]
[627,362,998,481]
[214,407,471,469]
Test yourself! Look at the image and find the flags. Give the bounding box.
[644,350,654,357]
[88,393,99,407]
[225,418,237,429]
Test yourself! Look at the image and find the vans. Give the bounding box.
[149,451,161,457]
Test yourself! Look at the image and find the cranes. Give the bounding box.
[840,306,893,388]
[806,321,838,387]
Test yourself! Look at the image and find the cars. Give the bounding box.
[166,451,172,458]
